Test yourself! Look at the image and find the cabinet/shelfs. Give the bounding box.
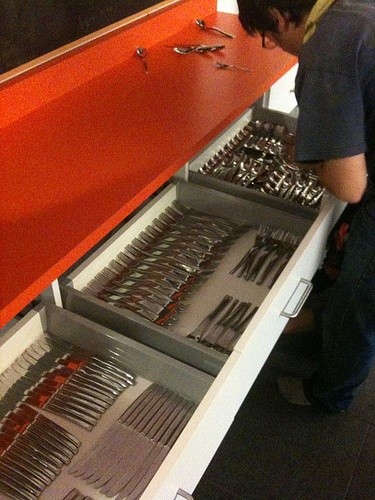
[0,0,348,500]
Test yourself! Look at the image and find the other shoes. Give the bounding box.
[277,377,314,405]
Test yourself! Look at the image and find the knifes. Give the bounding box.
[1,329,199,500]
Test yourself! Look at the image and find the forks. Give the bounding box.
[81,199,309,359]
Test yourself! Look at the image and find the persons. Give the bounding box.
[238,0,374,415]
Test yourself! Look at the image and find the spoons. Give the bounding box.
[173,44,226,55]
[136,47,149,74]
[200,118,324,209]
[195,18,236,38]
[216,62,252,73]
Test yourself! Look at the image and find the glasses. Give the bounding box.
[261,30,278,49]
